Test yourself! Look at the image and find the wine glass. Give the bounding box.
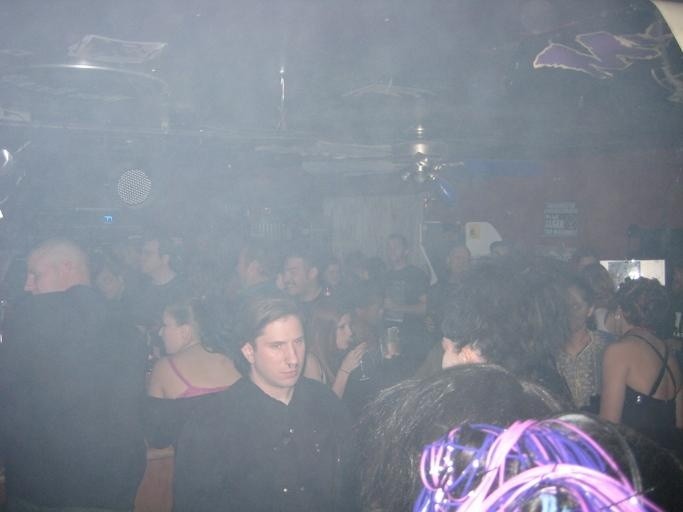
[348,338,372,381]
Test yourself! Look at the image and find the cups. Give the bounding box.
[378,333,402,358]
